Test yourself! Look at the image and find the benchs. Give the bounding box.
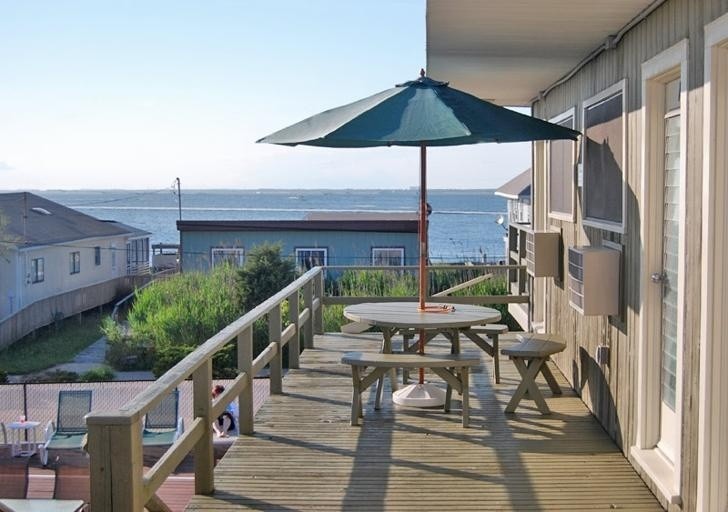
[340,352,479,428]
[500,333,568,415]
[392,323,508,384]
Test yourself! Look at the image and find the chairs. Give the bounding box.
[142,387,183,447]
[38,390,91,467]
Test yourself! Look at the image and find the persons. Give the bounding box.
[211,385,240,436]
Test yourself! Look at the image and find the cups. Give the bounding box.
[20,416,26,423]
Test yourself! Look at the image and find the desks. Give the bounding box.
[343,302,502,412]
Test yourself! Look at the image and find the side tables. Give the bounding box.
[7,422,40,459]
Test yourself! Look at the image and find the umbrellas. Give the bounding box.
[253,68,583,384]
